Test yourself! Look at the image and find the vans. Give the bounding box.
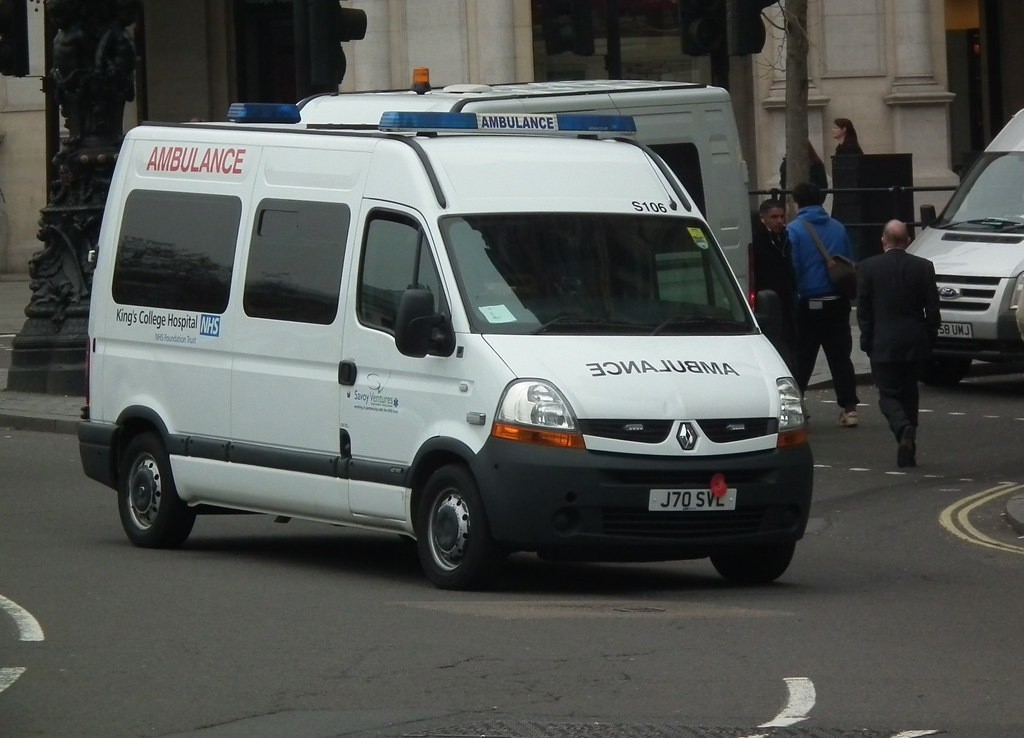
[905,106,1024,382]
[74,100,818,592]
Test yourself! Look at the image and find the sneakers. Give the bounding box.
[837,411,858,425]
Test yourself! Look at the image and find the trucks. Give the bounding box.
[293,68,757,318]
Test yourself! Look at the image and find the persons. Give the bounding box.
[856,218,942,468]
[752,199,801,348]
[784,183,860,427]
[779,139,828,207]
[46,0,141,148]
[831,118,864,155]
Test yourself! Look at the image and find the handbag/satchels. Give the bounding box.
[825,253,857,300]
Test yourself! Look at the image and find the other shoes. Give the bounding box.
[896,437,917,467]
